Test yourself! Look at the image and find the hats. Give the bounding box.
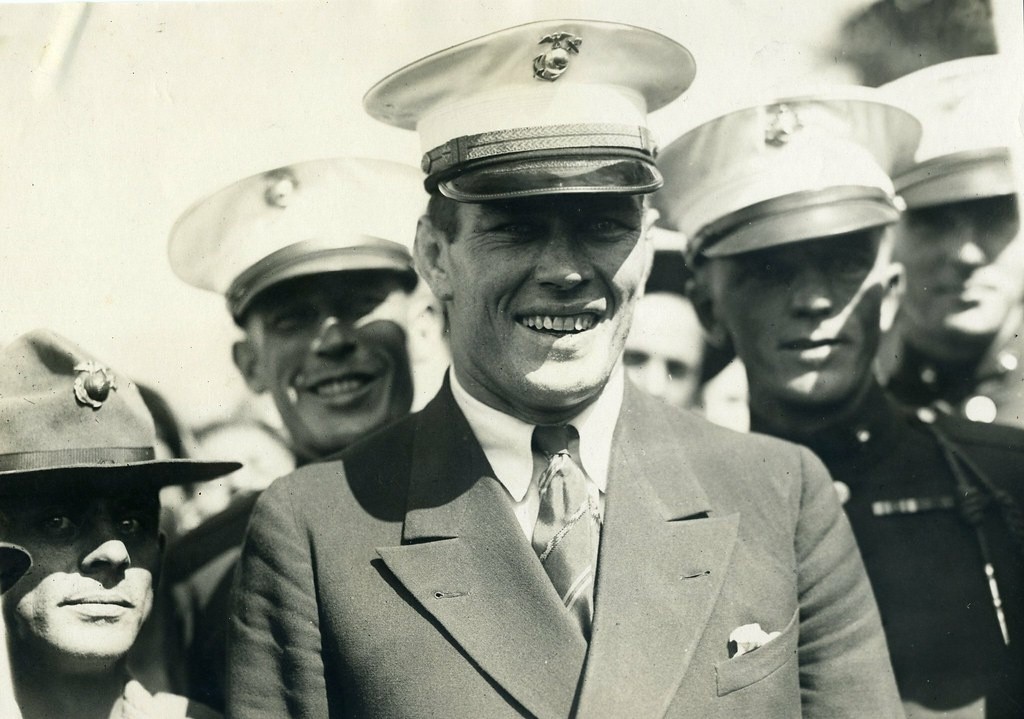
[168,152,440,320]
[361,20,697,203]
[873,54,1018,211]
[0,322,244,485]
[648,92,922,264]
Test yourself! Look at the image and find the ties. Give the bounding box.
[527,424,600,640]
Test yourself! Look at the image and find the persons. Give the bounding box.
[166,145,434,449]
[654,97,1024,717]
[0,330,225,719]
[601,228,738,419]
[875,56,1024,425]
[225,20,905,719]
